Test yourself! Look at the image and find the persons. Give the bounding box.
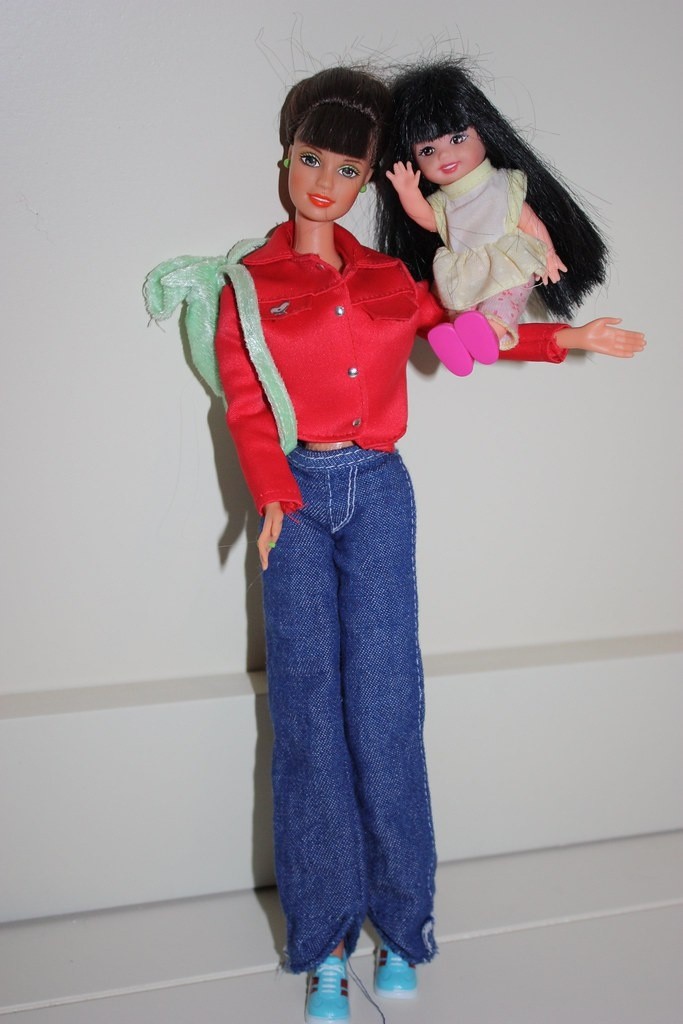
[214,67,648,1023]
[375,54,608,378]
